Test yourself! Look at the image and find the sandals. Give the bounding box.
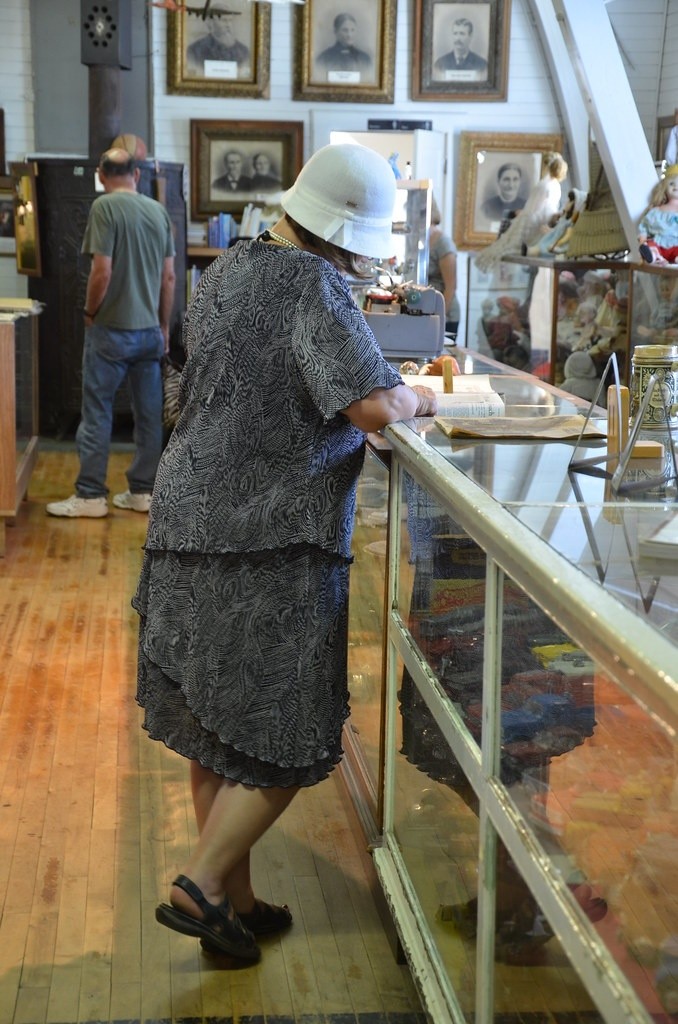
[200,897,293,951]
[438,890,523,938]
[494,887,607,957]
[156,874,260,965]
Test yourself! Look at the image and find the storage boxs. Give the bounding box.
[431,534,527,616]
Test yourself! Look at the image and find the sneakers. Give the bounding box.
[113,488,154,513]
[45,495,109,518]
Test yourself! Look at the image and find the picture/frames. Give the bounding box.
[455,131,564,250]
[10,162,42,278]
[190,119,302,222]
[167,0,272,99]
[292,0,397,103]
[412,0,511,103]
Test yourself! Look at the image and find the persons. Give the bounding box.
[45,148,176,517]
[402,445,608,964]
[132,139,439,964]
[477,152,678,405]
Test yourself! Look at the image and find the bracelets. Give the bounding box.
[84,307,94,317]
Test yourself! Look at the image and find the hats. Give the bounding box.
[280,140,398,260]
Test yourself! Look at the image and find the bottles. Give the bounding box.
[628,345,678,427]
